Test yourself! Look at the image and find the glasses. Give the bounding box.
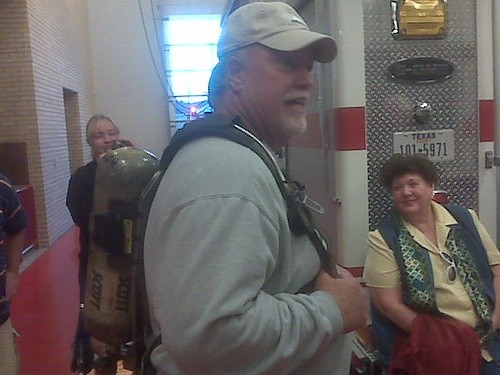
[439,252,457,285]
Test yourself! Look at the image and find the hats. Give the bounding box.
[217,1,336,63]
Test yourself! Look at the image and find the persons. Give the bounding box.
[364,153,500,375]
[66,114,133,351]
[0,174,25,375]
[141,2,368,375]
